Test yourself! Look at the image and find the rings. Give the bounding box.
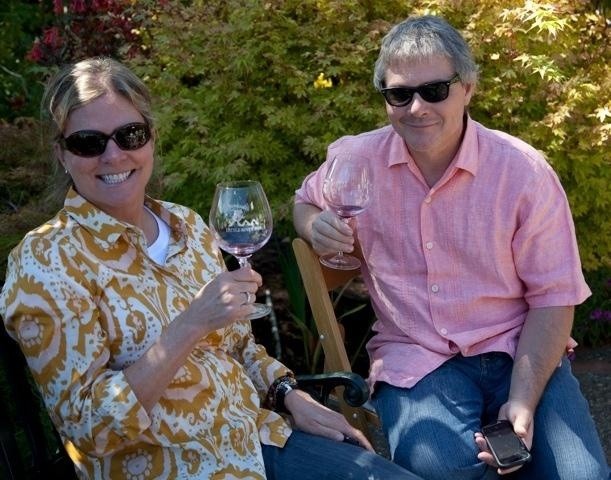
[245,292,251,304]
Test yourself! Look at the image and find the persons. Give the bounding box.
[0,58,428,480]
[293,14,609,480]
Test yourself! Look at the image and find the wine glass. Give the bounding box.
[319,154,374,270]
[209,181,274,320]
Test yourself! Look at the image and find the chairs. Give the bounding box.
[1,221,386,477]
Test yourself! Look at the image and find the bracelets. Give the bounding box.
[276,383,296,409]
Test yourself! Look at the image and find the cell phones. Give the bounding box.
[481,416,535,471]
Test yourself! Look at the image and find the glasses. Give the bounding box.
[55,122,151,158]
[381,73,459,107]
